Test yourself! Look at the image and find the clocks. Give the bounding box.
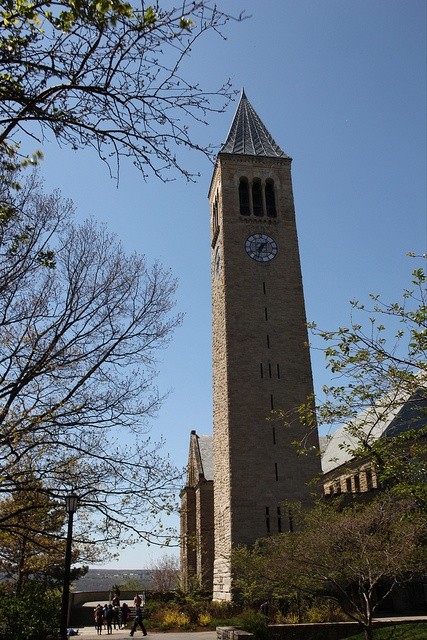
[244,233,277,262]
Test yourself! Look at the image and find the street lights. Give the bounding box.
[57,491,79,640]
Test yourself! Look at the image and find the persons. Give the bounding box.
[112,594,119,608]
[103,604,109,630]
[134,594,142,609]
[115,588,121,597]
[94,604,102,630]
[120,603,131,630]
[129,603,147,636]
[113,606,121,629]
[96,606,105,635]
[106,604,114,635]
